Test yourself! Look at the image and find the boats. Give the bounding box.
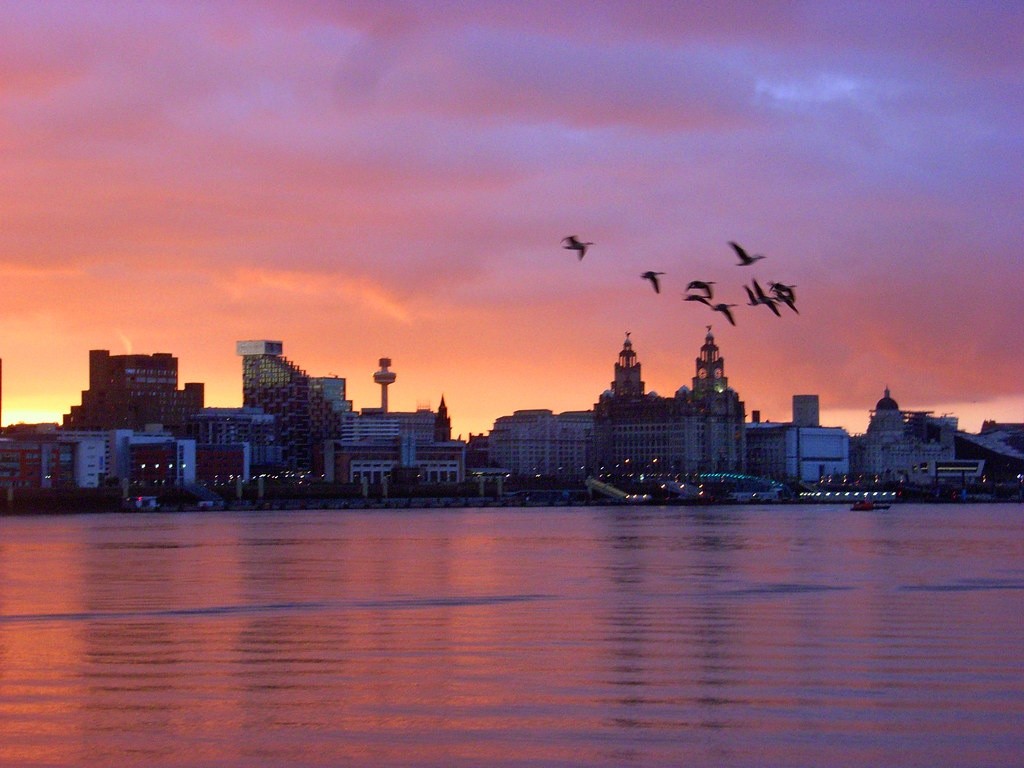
[851,500,892,510]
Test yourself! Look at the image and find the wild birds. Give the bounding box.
[683,294,714,308]
[641,271,666,293]
[686,280,716,299]
[561,236,593,261]
[714,303,739,326]
[743,279,799,318]
[728,241,765,266]
